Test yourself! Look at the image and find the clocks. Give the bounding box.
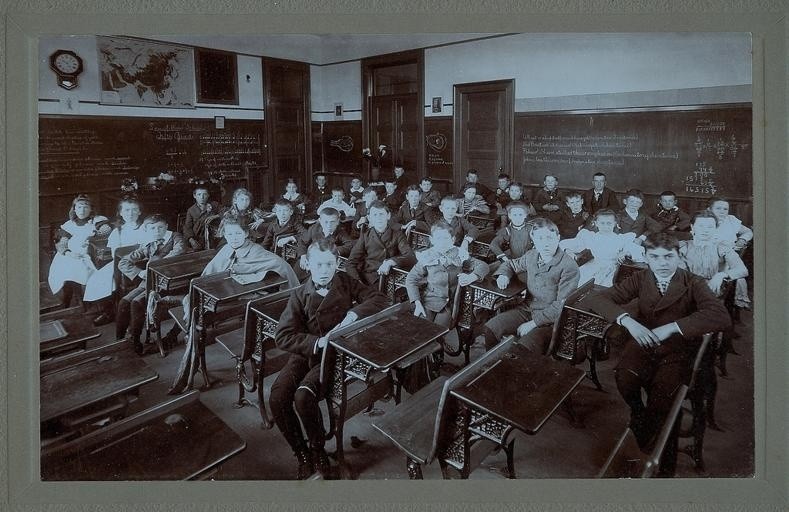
[47,48,86,91]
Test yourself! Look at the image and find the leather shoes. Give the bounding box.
[309,444,330,477]
[294,447,314,480]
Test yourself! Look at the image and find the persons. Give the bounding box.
[482,217,581,357]
[587,228,733,477]
[47,194,110,314]
[404,220,492,330]
[83,196,154,326]
[115,214,186,355]
[269,236,394,481]
[146,213,302,356]
[180,163,755,312]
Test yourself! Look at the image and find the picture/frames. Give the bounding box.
[430,95,444,116]
[214,114,226,130]
[95,32,198,112]
[334,102,343,118]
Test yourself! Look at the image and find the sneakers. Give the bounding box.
[93,312,114,326]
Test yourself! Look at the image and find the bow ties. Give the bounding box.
[314,283,333,290]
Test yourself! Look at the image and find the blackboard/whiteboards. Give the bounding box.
[513,102,752,202]
[38,113,265,197]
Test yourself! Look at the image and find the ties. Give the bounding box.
[656,282,669,296]
